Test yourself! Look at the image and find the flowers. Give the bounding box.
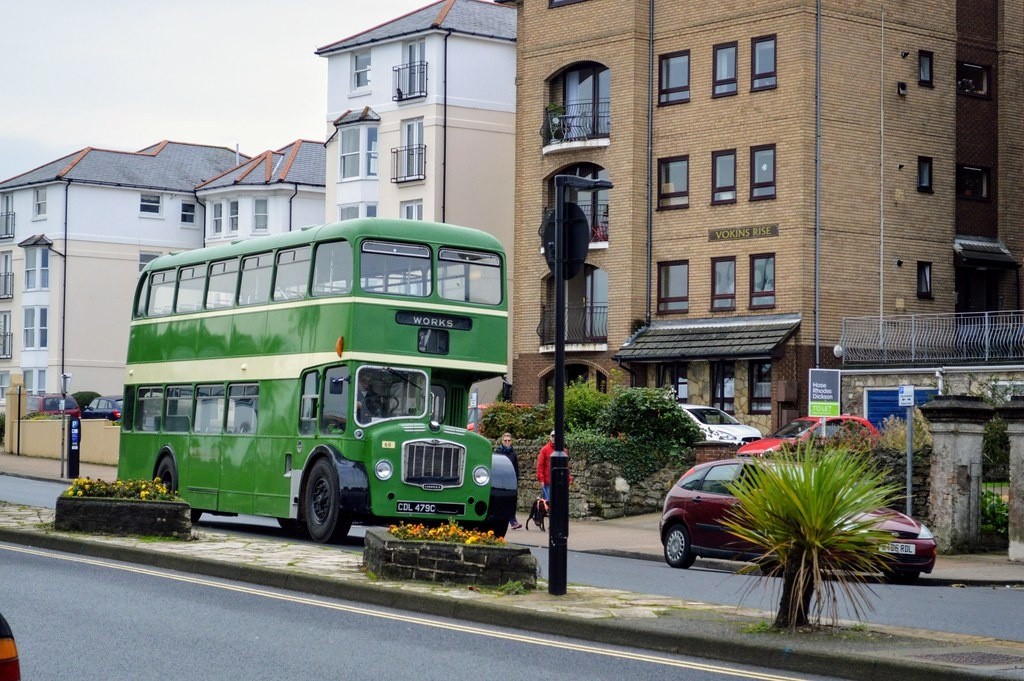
[65,474,181,502]
[387,515,507,546]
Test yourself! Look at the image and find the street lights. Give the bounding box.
[548,174,615,595]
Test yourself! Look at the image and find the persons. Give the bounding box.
[537,430,573,522]
[340,374,384,421]
[494,432,522,532]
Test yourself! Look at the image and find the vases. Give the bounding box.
[54,496,193,540]
[363,528,537,590]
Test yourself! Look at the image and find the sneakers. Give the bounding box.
[510,523,522,529]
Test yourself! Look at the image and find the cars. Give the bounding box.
[659,456,936,585]
[26,393,81,420]
[678,403,763,446]
[81,394,124,421]
[736,412,882,463]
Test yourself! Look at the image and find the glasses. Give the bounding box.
[503,439,511,442]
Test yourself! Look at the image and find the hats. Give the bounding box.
[551,430,555,435]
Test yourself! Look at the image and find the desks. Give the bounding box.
[556,115,582,142]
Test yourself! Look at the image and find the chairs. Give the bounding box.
[591,211,608,242]
[547,109,591,143]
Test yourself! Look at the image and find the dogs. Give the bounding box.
[526,498,549,532]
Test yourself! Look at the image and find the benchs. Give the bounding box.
[151,285,343,315]
[154,414,192,432]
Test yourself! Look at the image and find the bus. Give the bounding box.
[117,217,515,546]
